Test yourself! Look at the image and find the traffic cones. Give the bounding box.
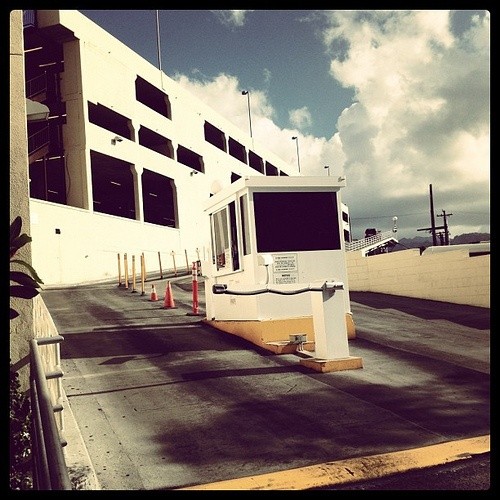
[160,280,179,309]
[148,284,159,303]
